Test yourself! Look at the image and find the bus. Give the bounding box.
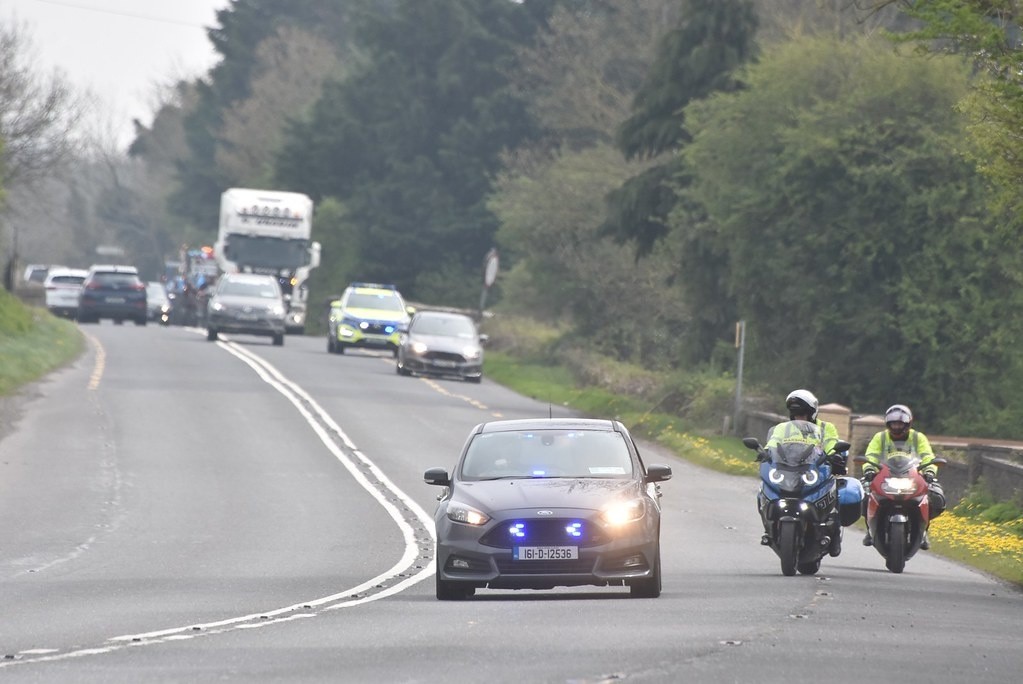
[214,186,323,332]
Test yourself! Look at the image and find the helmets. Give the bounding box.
[786,389,819,422]
[885,404,913,428]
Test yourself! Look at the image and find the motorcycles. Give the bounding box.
[852,453,948,575]
[741,418,866,576]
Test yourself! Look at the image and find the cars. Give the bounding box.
[197,271,291,345]
[398,304,491,382]
[324,281,415,360]
[144,278,173,322]
[423,417,674,602]
[24,263,67,290]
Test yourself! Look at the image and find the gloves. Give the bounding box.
[757,449,772,464]
[831,454,846,476]
[923,470,934,483]
[864,469,876,482]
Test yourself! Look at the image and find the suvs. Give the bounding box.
[41,267,88,320]
[76,263,147,325]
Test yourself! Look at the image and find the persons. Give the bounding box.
[757,389,842,557]
[863,404,938,550]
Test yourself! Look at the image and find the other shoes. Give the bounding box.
[920,531,930,550]
[830,545,841,556]
[863,534,874,546]
[761,532,770,545]
